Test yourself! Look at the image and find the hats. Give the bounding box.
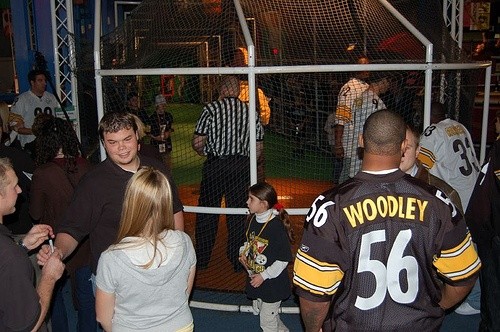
[154,94,166,107]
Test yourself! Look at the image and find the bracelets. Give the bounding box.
[17,239,30,254]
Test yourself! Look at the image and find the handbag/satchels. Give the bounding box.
[325,113,336,144]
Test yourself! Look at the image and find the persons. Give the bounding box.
[239,182,292,332]
[292,110,482,332]
[0,31,500,332]
